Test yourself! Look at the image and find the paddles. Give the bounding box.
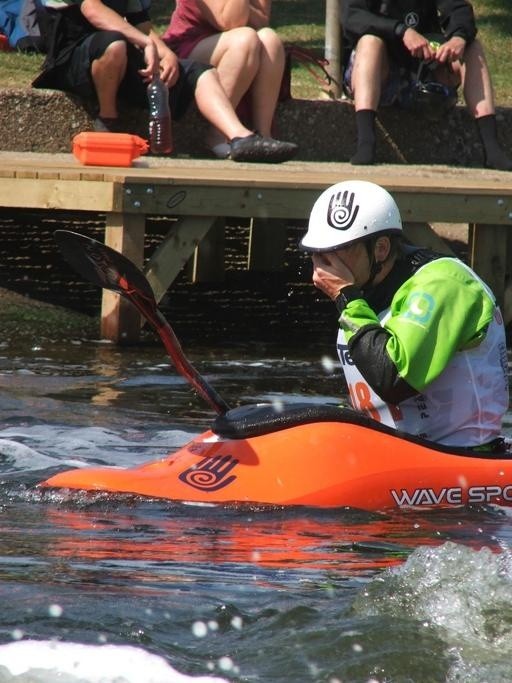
[53,229,231,415]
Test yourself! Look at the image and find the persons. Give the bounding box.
[294,178,511,457]
[339,0,512,171]
[161,0,288,158]
[31,0,299,164]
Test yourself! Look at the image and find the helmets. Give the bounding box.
[299,180,402,252]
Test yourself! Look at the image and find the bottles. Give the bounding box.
[146,71,174,154]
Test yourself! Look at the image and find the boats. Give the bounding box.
[32,396,512,515]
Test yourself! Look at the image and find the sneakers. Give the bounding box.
[226,131,299,163]
